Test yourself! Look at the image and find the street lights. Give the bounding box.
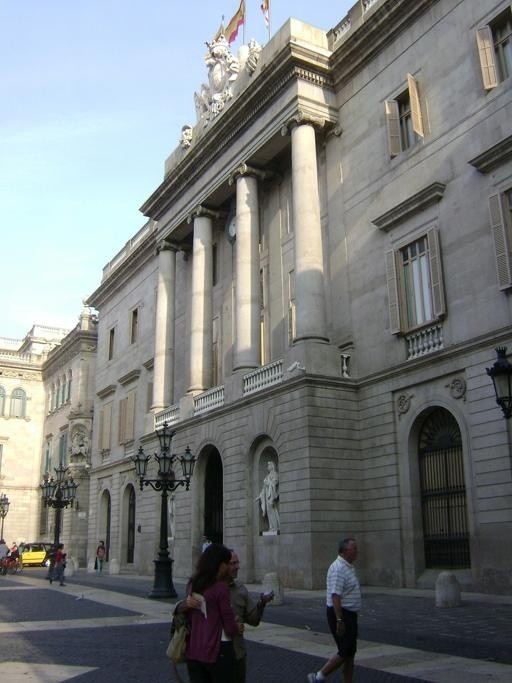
[483,345,511,466]
[129,420,199,597]
[0,492,11,539]
[39,462,80,580]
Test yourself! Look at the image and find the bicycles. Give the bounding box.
[1,556,23,575]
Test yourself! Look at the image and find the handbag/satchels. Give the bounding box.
[166,623,189,663]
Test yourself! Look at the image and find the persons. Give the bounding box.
[254,460,281,534]
[304,536,365,683]
[49,542,69,588]
[1,539,26,575]
[201,535,212,553]
[95,540,106,573]
[171,548,275,683]
[187,546,245,681]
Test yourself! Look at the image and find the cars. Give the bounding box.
[16,542,54,566]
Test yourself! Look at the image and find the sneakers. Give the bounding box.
[307,672,327,683]
